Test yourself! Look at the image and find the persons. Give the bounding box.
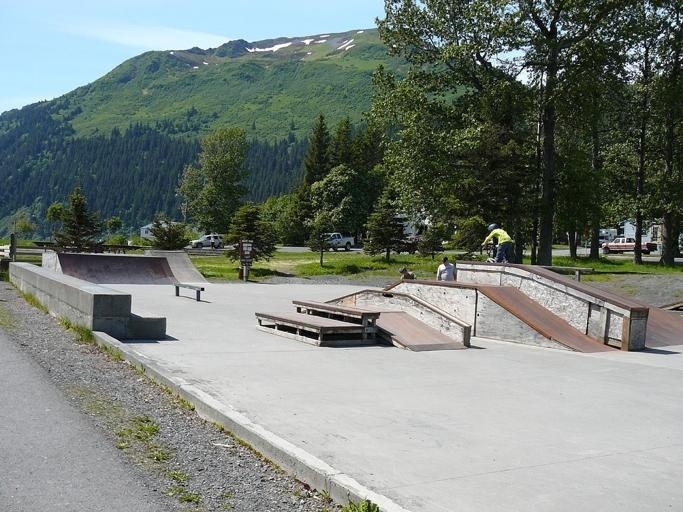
[436,256,456,281]
[398,267,415,280]
[481,223,514,264]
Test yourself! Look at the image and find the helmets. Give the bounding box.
[487,223,497,231]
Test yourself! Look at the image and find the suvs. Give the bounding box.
[191,235,225,248]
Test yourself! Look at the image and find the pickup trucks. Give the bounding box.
[602,238,657,255]
[320,232,355,252]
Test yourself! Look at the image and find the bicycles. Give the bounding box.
[481,244,507,264]
